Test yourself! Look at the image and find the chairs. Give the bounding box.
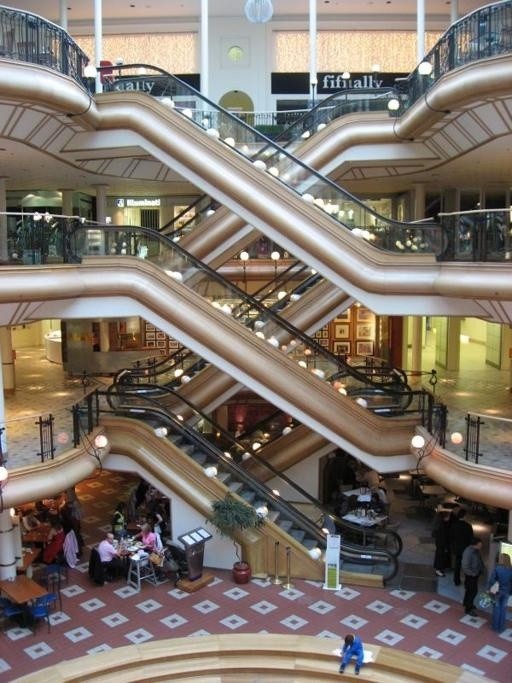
[92,547,117,582]
[29,596,51,635]
[127,553,157,592]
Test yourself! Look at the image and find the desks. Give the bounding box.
[0,499,59,628]
[109,486,166,580]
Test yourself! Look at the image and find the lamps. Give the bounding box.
[140,209,368,412]
[32,211,60,229]
[0,466,10,512]
[308,547,323,562]
[92,359,293,518]
[411,430,465,453]
[161,57,434,250]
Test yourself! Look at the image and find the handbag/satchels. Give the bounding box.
[477,591,498,609]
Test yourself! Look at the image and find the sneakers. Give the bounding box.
[338,664,361,675]
[463,602,477,617]
[435,569,463,587]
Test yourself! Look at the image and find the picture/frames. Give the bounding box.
[356,309,376,356]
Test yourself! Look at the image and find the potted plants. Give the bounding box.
[204,492,265,585]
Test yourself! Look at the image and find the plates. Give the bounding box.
[127,547,139,551]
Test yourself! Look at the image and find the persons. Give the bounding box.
[446,509,474,585]
[487,552,512,633]
[447,504,461,520]
[339,633,364,675]
[460,537,485,616]
[22,477,170,587]
[428,511,445,577]
[341,459,360,488]
[340,494,358,517]
[360,467,379,489]
[365,492,385,543]
